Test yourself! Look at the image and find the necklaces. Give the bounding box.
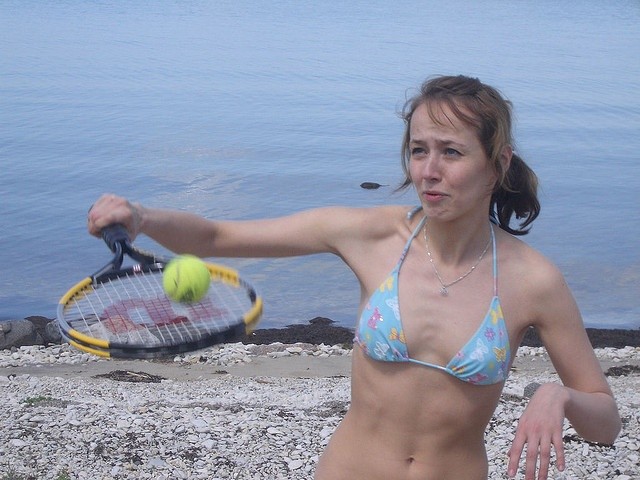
[424,222,492,297]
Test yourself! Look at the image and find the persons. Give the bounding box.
[87,76,621,480]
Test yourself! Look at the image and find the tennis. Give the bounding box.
[161,253,210,303]
